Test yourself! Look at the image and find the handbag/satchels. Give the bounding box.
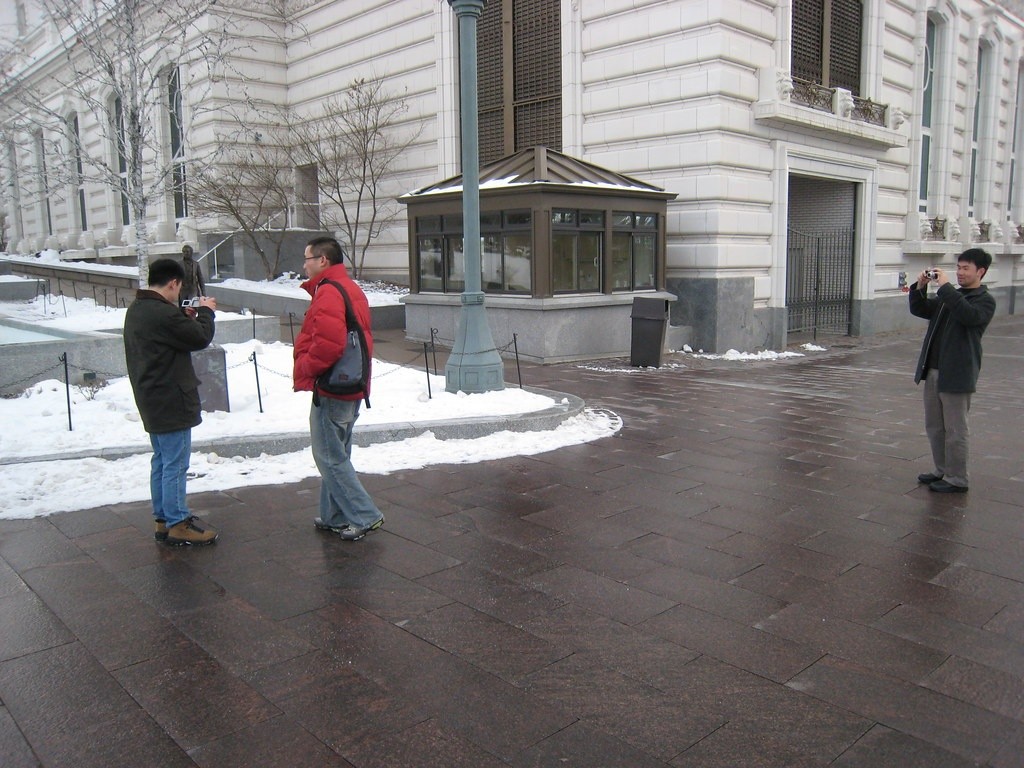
[312,279,370,408]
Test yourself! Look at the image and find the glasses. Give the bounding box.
[301,255,330,265]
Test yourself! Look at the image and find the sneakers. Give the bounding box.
[167,515,219,546]
[929,480,968,493]
[918,472,942,483]
[340,513,386,541]
[155,519,169,541]
[314,518,350,532]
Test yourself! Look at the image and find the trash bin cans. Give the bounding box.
[629,296,672,367]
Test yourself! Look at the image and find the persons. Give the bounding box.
[293,237,385,541]
[176,245,206,308]
[123,259,219,546]
[909,248,996,493]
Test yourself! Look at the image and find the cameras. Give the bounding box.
[924,270,938,279]
[181,296,209,308]
